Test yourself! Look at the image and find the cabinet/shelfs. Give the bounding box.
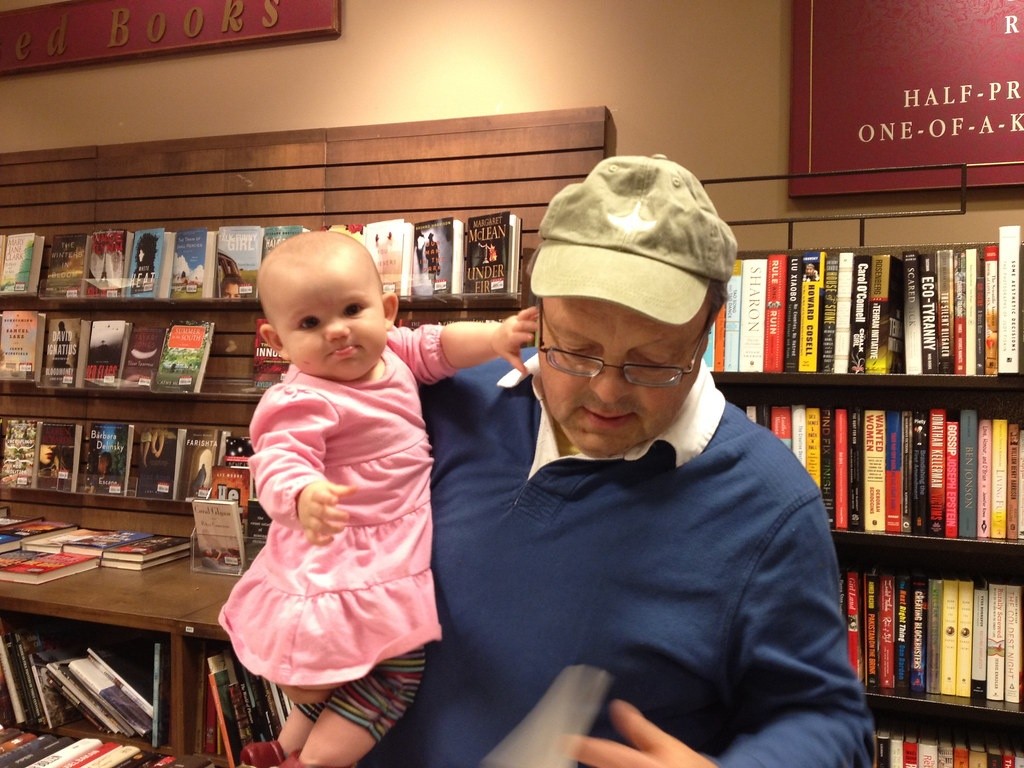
[710,369,1024,726]
[0,104,610,768]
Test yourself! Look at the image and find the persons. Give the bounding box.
[216,231,537,768]
[355,153,876,768]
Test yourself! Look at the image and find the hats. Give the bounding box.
[526,154,738,325]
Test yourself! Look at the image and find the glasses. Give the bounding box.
[539,296,708,387]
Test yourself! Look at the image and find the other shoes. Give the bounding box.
[240,741,304,768]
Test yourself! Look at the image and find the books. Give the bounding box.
[700,226,1024,767]
[0,211,523,587]
[0,630,296,768]
